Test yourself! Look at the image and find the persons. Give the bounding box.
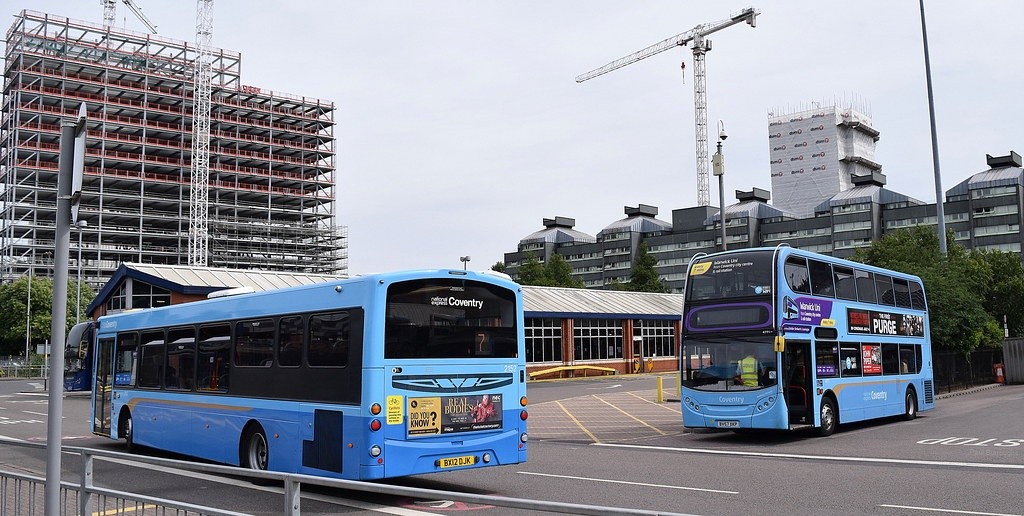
[818,279,834,296]
[734,346,767,387]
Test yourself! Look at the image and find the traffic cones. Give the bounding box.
[995,364,1005,384]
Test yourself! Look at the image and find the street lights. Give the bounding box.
[716,119,728,252]
[459,255,471,270]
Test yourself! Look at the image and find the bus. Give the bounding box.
[679,243,934,438]
[65,321,97,391]
[94,268,529,488]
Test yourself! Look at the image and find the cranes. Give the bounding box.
[576,6,762,210]
